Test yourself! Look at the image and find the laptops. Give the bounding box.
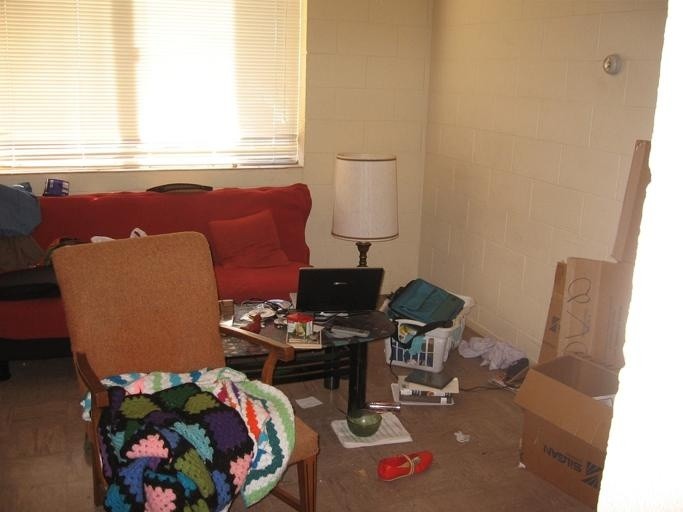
[289,267,385,312]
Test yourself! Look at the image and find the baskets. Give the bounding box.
[380,291,473,374]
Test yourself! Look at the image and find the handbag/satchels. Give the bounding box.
[391,278,464,328]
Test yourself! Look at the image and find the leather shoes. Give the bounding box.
[377,451,432,482]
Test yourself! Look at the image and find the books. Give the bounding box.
[285,322,324,351]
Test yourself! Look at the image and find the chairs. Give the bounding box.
[47,229,321,512]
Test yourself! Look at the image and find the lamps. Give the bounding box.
[330,151,401,266]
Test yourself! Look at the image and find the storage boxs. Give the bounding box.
[500,352,620,512]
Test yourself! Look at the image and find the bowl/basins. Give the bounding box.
[346,409,382,436]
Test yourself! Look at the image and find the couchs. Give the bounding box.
[1,182,314,382]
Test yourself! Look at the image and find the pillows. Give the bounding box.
[208,208,291,270]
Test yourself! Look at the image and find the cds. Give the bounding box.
[249,308,275,317]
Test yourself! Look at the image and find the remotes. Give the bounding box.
[331,325,370,338]
